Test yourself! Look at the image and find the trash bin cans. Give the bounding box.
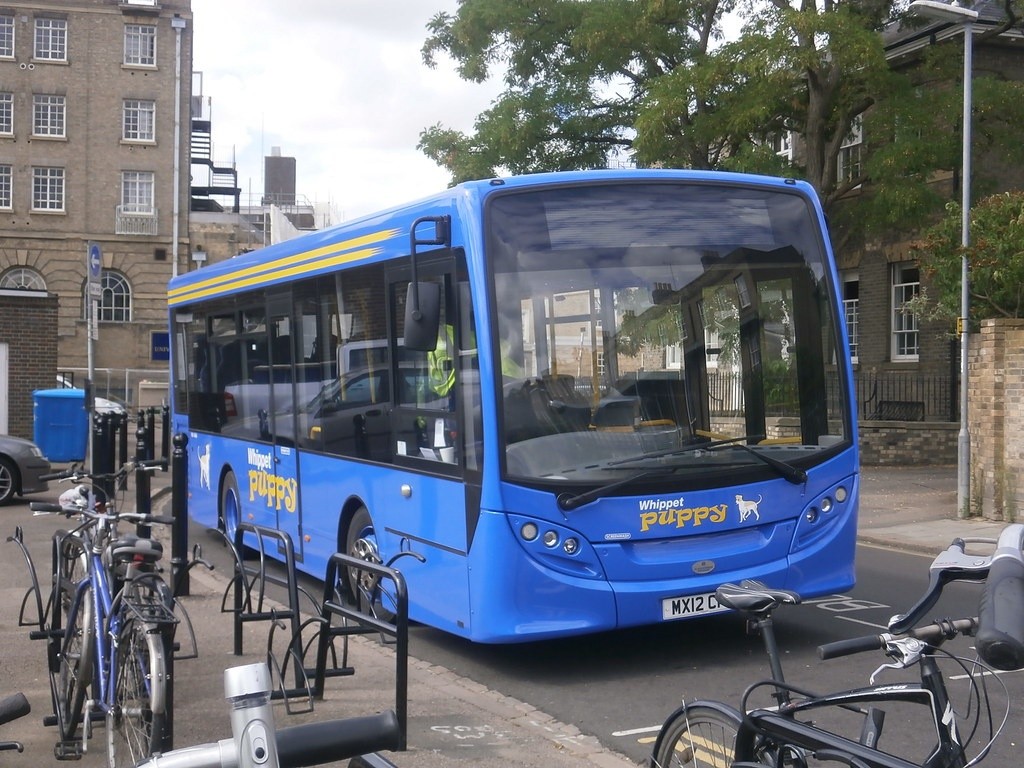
[33,388,88,463]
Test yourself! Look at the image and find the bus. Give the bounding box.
[169,168,861,647]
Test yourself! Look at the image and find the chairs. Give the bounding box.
[195,332,353,396]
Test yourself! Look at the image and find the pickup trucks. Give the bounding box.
[225,339,480,429]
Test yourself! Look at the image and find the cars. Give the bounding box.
[220,361,523,472]
[57,375,127,433]
[431,373,682,481]
[0,433,53,507]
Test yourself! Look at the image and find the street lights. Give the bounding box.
[909,0,980,516]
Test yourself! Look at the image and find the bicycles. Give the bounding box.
[650,523,1023,768]
[30,460,175,768]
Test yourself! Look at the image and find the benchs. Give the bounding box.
[867,400,925,421]
